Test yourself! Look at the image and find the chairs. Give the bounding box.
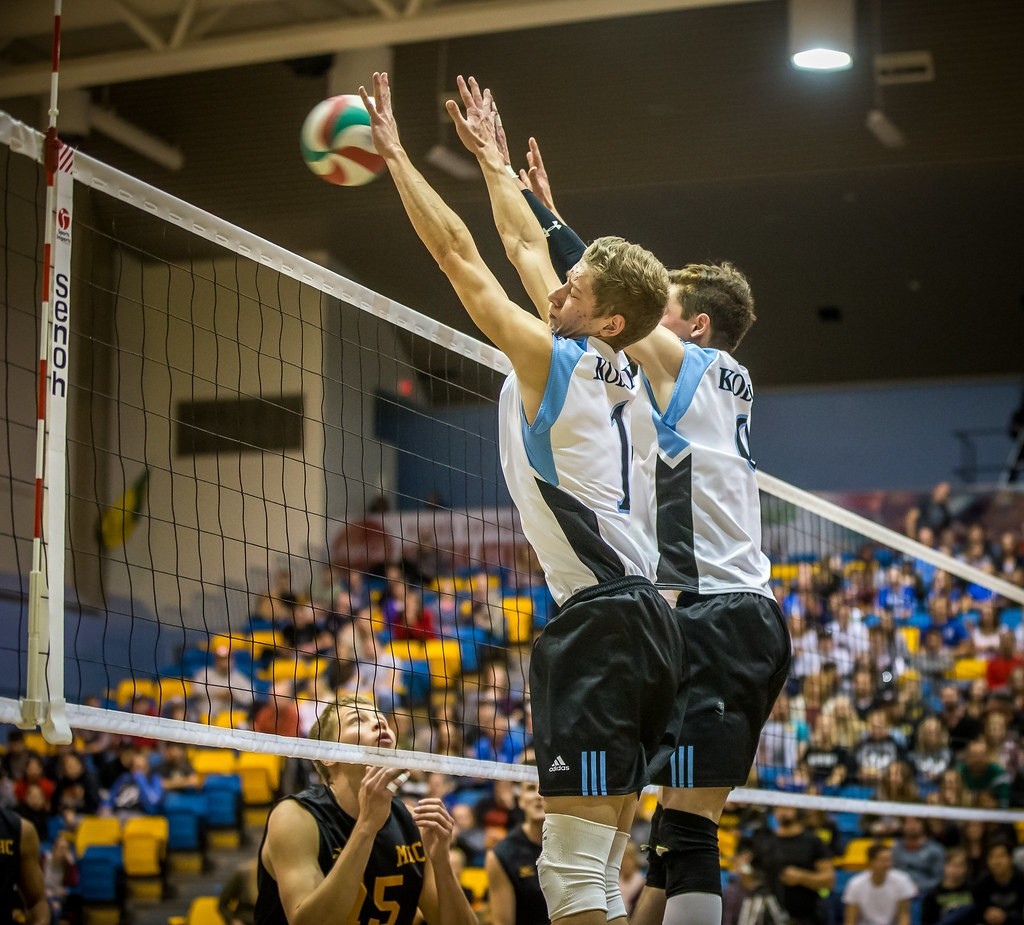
[0,547,1024,925]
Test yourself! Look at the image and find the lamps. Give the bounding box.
[786,0,860,74]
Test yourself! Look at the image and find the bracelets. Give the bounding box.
[505,164,519,178]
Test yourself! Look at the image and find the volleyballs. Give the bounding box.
[298,94,386,188]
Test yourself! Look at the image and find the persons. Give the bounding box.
[254,697,480,925]
[1009,384,1024,484]
[492,101,791,925]
[358,71,692,925]
[1,481,1024,925]
